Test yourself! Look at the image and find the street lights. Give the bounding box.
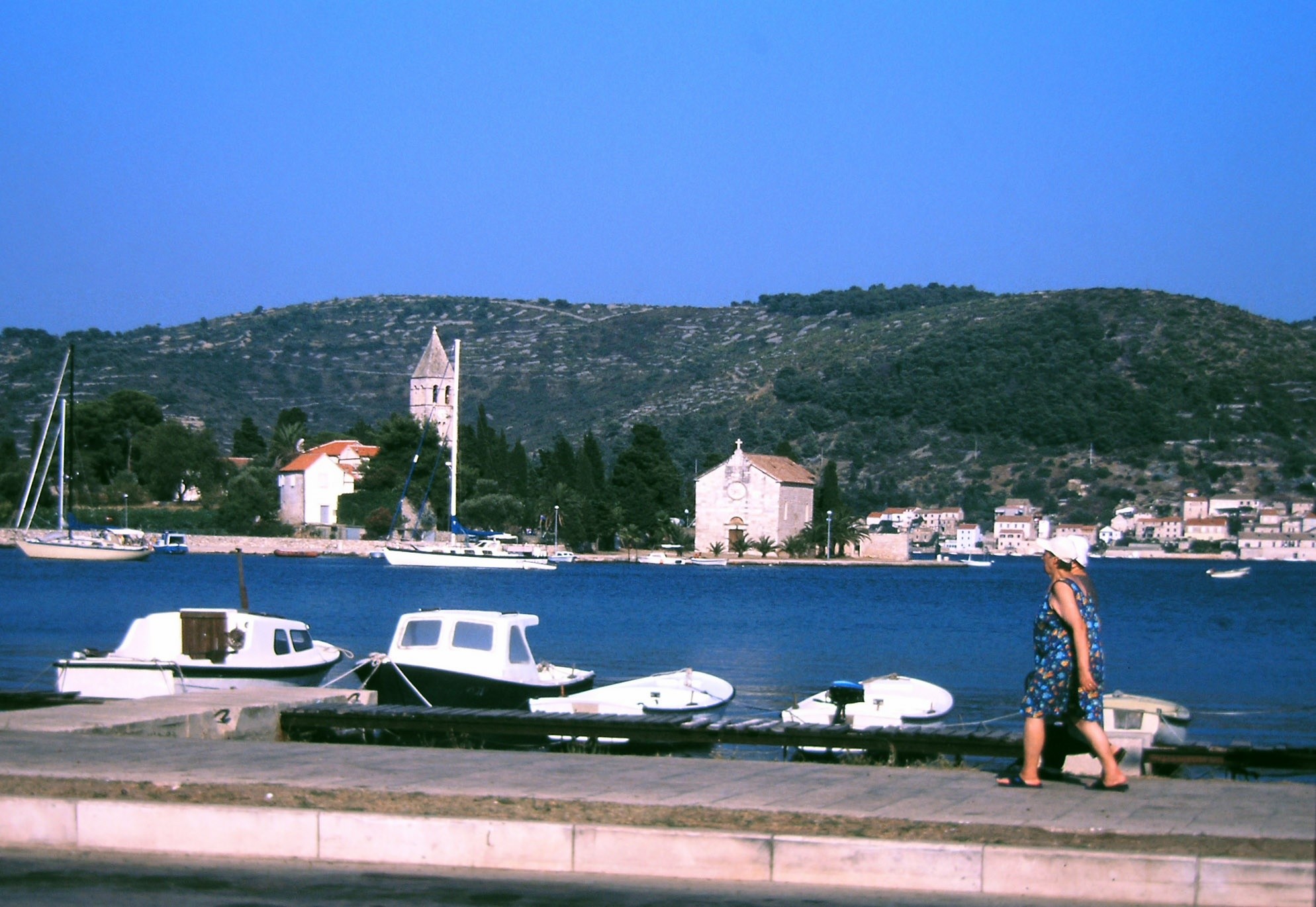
[552,505,559,551]
[827,510,833,558]
[684,508,690,526]
[124,492,130,528]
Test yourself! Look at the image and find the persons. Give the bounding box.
[993,534,1131,792]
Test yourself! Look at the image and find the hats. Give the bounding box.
[1036,537,1077,563]
[1067,535,1090,567]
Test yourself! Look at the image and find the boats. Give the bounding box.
[147,531,189,555]
[1035,689,1193,779]
[522,561,558,572]
[961,550,993,567]
[345,609,600,754]
[635,550,687,566]
[774,673,956,762]
[1204,566,1252,580]
[690,552,727,566]
[547,550,580,563]
[988,551,1022,557]
[275,547,320,558]
[526,666,736,758]
[47,551,358,707]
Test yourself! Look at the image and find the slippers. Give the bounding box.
[1087,778,1129,791]
[1100,748,1126,780]
[997,774,1043,787]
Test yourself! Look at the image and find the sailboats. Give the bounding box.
[1,342,154,560]
[359,338,560,568]
